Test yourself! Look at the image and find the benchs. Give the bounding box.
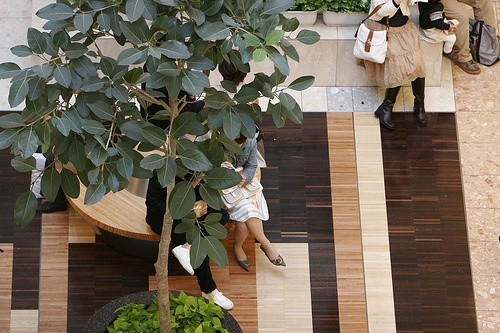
[53,154,162,257]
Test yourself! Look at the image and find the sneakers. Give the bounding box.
[172,245,194,276]
[202,288,234,311]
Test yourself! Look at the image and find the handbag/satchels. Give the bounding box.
[218,162,264,209]
[469,18,499,67]
[352,1,390,64]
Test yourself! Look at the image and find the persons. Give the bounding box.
[145,133,286,311]
[357,0,428,129]
[140,34,259,130]
[418,0,496,73]
[33,88,122,213]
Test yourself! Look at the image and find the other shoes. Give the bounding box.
[233,244,250,272]
[36,201,67,213]
[453,59,481,73]
[442,44,460,60]
[260,244,286,267]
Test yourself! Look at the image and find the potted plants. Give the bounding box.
[0,0,321,333]
[277,0,322,26]
[322,0,372,27]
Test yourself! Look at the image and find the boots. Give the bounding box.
[374,85,401,130]
[411,77,427,126]
